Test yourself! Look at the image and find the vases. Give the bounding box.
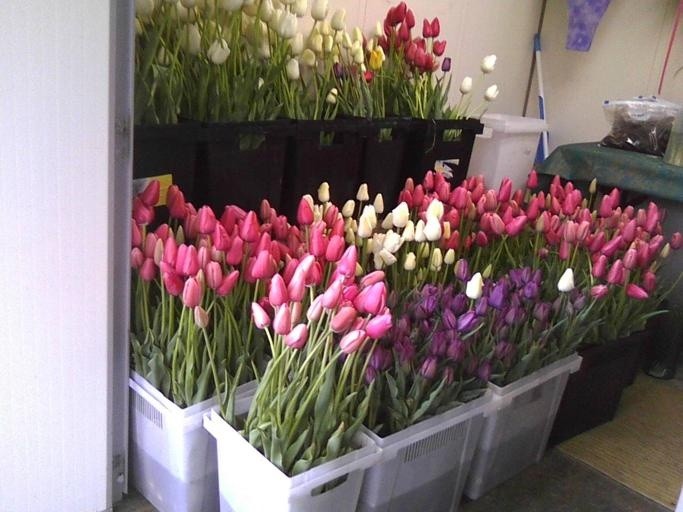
[128,352,580,512]
